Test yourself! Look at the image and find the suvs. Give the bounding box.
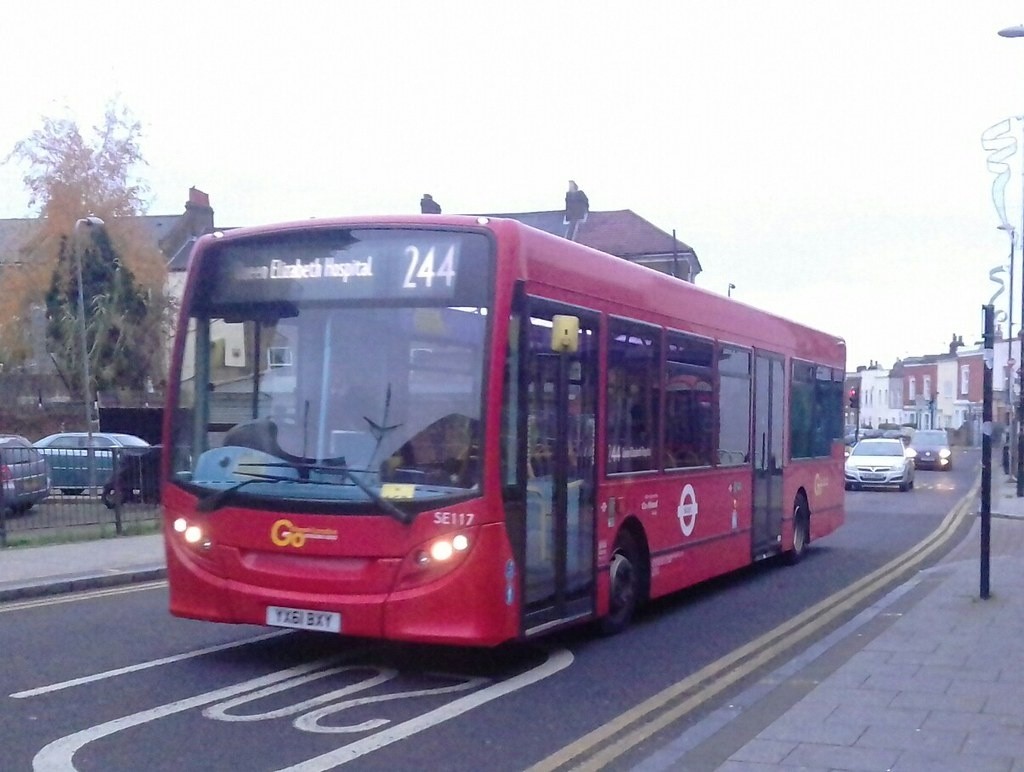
[908,428,955,471]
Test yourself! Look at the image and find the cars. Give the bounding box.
[844,437,918,493]
[0,433,54,514]
[101,444,189,511]
[845,426,915,448]
[32,432,152,496]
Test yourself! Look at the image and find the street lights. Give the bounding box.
[74,216,105,526]
[997,224,1016,475]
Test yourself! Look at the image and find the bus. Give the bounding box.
[159,212,846,652]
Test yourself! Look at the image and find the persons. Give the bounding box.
[384,411,448,470]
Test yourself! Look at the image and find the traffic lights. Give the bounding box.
[848,388,859,408]
